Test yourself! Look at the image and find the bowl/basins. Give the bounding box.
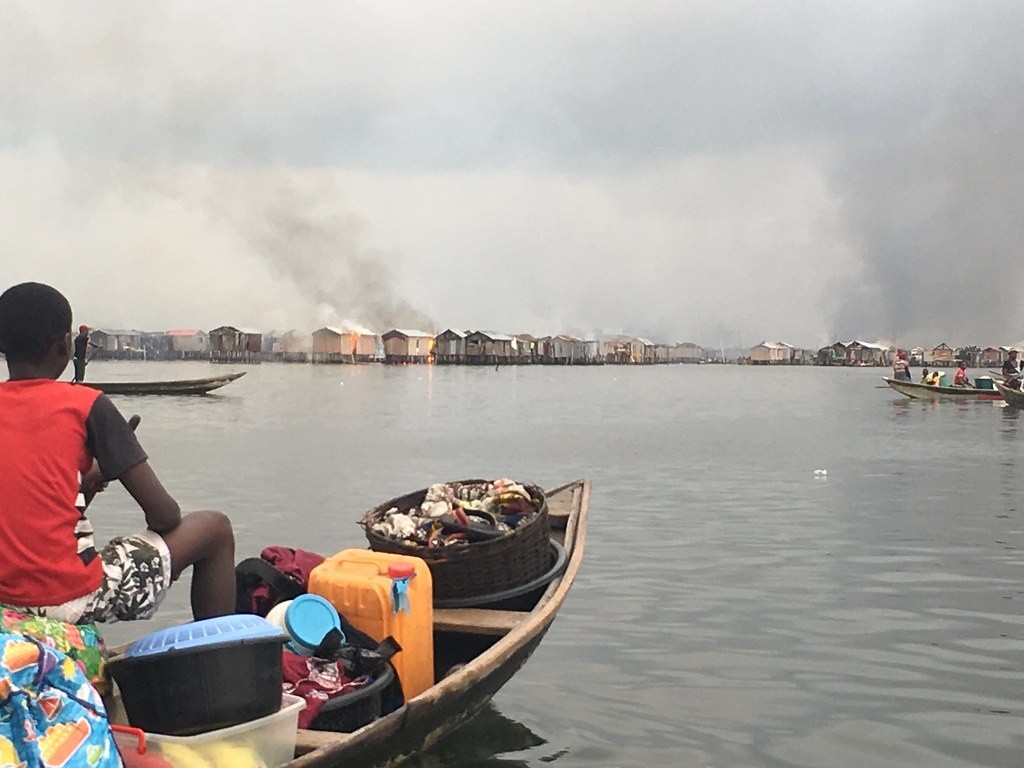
[101,633,291,738]
[434,537,568,610]
[311,661,395,732]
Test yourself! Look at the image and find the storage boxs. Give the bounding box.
[112,692,307,768]
[106,612,288,737]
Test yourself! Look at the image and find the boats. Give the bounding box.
[881,373,1007,402]
[991,375,1024,409]
[75,371,247,395]
[104,475,594,768]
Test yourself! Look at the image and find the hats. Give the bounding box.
[1008,348,1018,355]
[896,351,906,358]
[79,326,92,332]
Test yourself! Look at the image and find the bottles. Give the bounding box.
[261,592,345,656]
[814,469,826,475]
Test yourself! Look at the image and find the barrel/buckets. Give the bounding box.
[306,547,434,703]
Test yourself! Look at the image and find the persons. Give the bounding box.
[894,348,1024,391]
[0,282,238,626]
[72,325,92,383]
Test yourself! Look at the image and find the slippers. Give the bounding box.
[440,507,507,538]
